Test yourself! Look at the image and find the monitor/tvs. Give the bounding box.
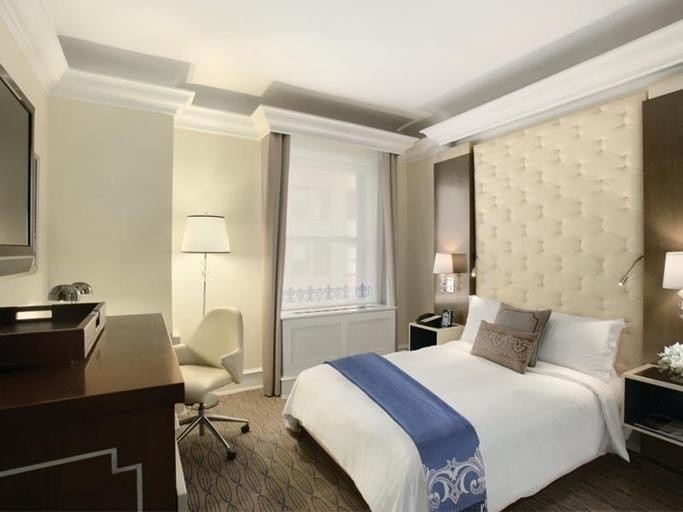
[0,64,38,279]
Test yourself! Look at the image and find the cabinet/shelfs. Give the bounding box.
[0,303,190,511]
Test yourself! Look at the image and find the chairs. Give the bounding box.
[173,308,249,459]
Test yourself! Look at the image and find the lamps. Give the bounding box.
[663,251,683,292]
[180,215,231,410]
[432,251,468,275]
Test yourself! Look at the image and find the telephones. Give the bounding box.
[415,311,442,328]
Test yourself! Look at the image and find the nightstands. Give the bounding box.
[409,321,465,350]
[620,362,681,478]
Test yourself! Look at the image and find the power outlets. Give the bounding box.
[446,278,454,293]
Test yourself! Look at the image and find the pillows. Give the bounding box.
[538,312,623,378]
[494,302,552,368]
[470,319,540,374]
[460,295,501,348]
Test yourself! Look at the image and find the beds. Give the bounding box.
[284,339,630,511]
